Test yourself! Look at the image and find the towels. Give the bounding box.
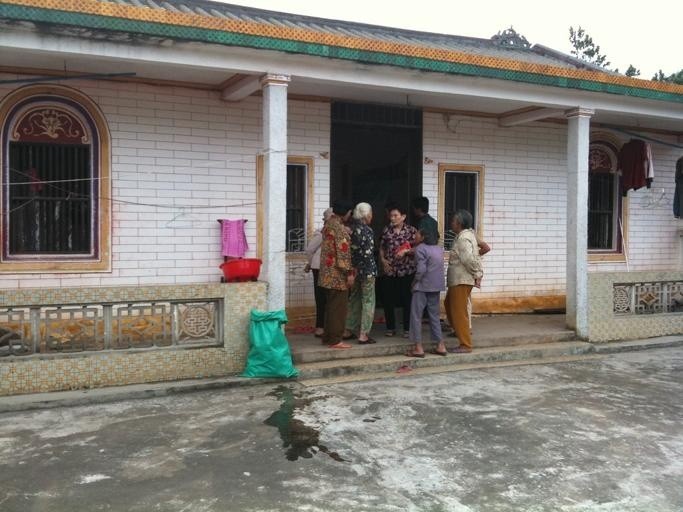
[220,219,249,259]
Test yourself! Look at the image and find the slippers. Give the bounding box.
[313,320,472,358]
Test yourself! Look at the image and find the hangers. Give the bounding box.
[625,135,652,147]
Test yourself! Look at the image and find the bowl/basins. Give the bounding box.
[218,258,263,281]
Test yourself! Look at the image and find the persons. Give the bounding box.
[373,200,397,304]
[405,227,448,357]
[411,196,440,244]
[379,204,417,338]
[443,209,490,353]
[303,196,379,349]
